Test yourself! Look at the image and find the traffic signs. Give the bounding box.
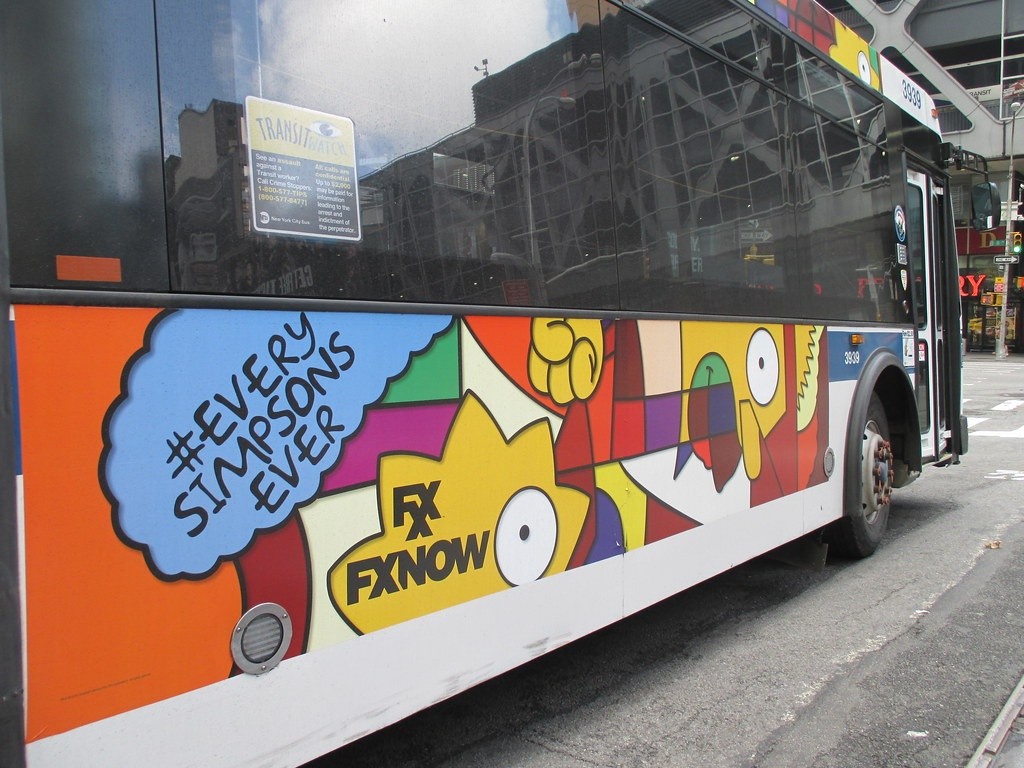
[993,256,1020,264]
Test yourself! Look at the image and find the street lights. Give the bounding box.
[1001,101,1021,361]
[521,96,576,311]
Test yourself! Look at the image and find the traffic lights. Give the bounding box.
[1014,234,1022,253]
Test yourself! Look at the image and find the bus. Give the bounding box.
[1,0,1002,768]
